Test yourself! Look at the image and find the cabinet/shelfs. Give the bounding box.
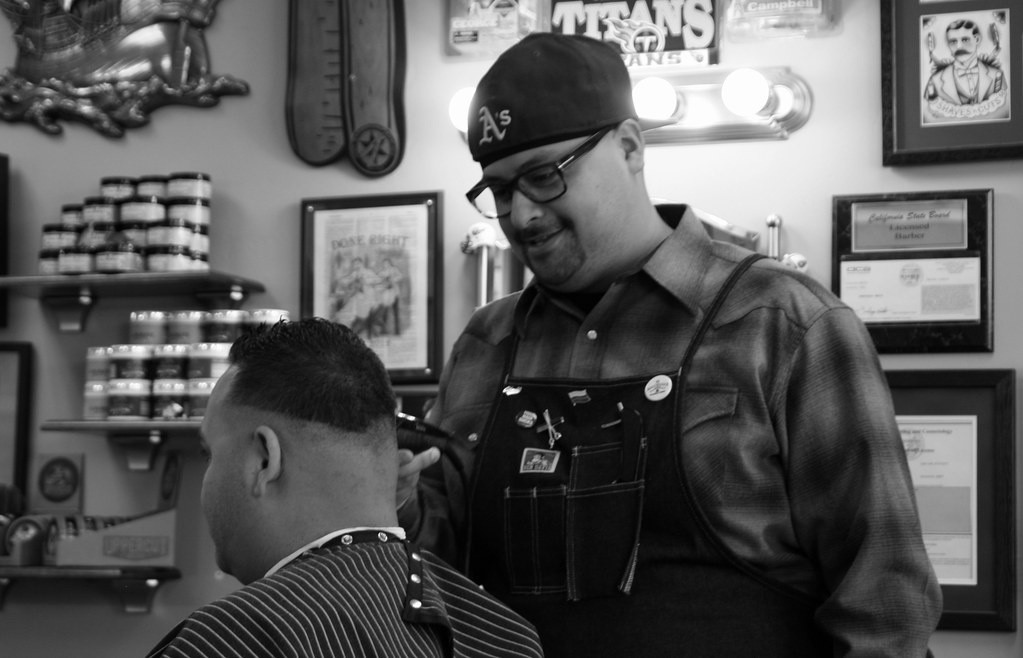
[0,273,266,578]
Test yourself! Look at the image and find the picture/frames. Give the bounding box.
[885,367,1018,630]
[879,0,1023,166]
[298,190,445,383]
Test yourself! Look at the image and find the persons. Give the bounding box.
[395,29,947,658]
[344,258,407,341]
[142,314,546,658]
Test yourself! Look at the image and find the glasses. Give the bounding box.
[463,124,619,220]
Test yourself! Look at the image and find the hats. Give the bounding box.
[466,31,640,166]
